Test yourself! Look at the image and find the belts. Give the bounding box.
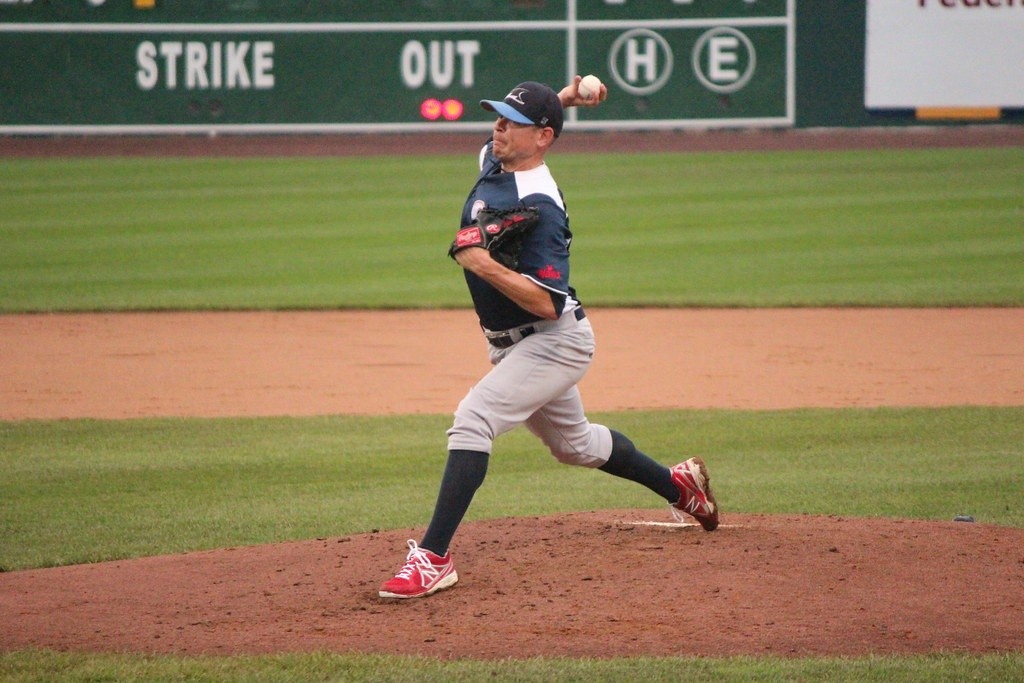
[485,306,586,347]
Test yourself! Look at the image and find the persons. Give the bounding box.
[378,75,718,599]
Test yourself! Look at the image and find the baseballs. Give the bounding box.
[579,74,602,100]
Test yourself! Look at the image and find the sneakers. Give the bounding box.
[378,539,459,598]
[669,454,719,531]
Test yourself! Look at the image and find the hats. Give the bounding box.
[480,81,563,136]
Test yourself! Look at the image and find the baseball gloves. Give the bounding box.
[445,205,541,271]
